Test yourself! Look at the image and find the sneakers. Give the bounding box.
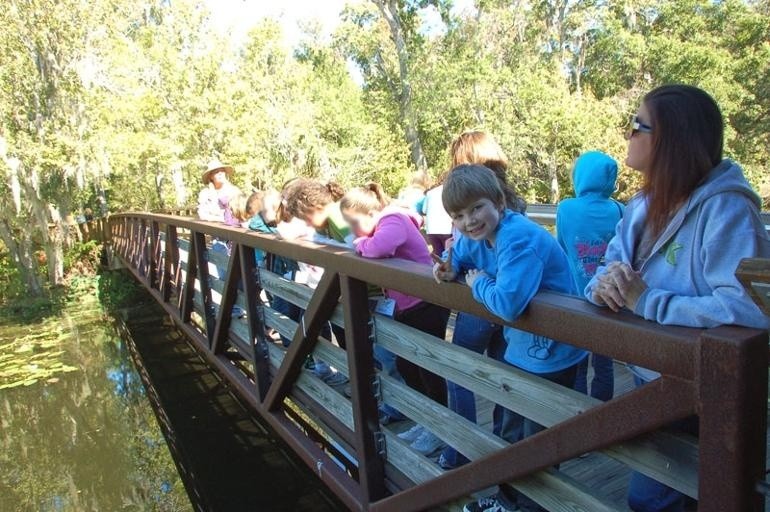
[307,361,351,400]
[378,403,460,469]
[462,494,506,511]
[231,305,245,319]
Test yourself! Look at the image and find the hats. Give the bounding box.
[202,158,234,184]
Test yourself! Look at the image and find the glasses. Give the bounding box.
[627,114,651,139]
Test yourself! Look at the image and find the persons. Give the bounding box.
[556,148,629,459]
[426,161,589,511]
[584,82,768,511]
[199,130,528,487]
